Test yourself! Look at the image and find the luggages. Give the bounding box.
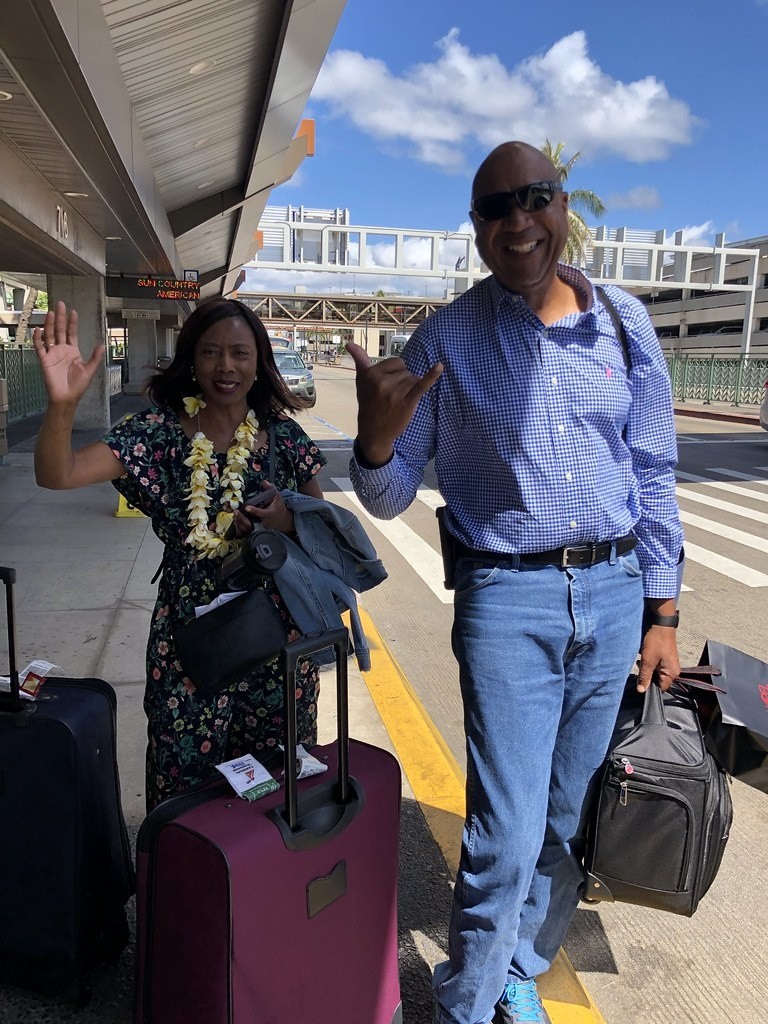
[0,566,137,1008]
[135,625,405,1024]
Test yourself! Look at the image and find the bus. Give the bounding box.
[307,334,341,357]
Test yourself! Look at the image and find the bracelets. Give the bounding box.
[653,611,680,628]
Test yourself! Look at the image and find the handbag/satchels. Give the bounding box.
[167,578,290,685]
[673,639,768,794]
[575,669,734,918]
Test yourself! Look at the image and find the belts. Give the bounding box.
[457,532,638,568]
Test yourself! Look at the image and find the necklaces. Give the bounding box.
[183,393,260,560]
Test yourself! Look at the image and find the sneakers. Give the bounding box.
[495,979,552,1024]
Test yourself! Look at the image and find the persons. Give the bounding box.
[33,297,329,817]
[344,142,686,1024]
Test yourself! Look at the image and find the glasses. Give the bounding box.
[470,180,564,222]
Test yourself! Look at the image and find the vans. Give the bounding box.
[392,335,412,351]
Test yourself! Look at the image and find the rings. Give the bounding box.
[47,344,54,348]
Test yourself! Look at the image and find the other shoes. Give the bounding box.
[432,997,493,1024]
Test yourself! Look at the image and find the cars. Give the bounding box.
[268,336,293,351]
[272,349,316,408]
